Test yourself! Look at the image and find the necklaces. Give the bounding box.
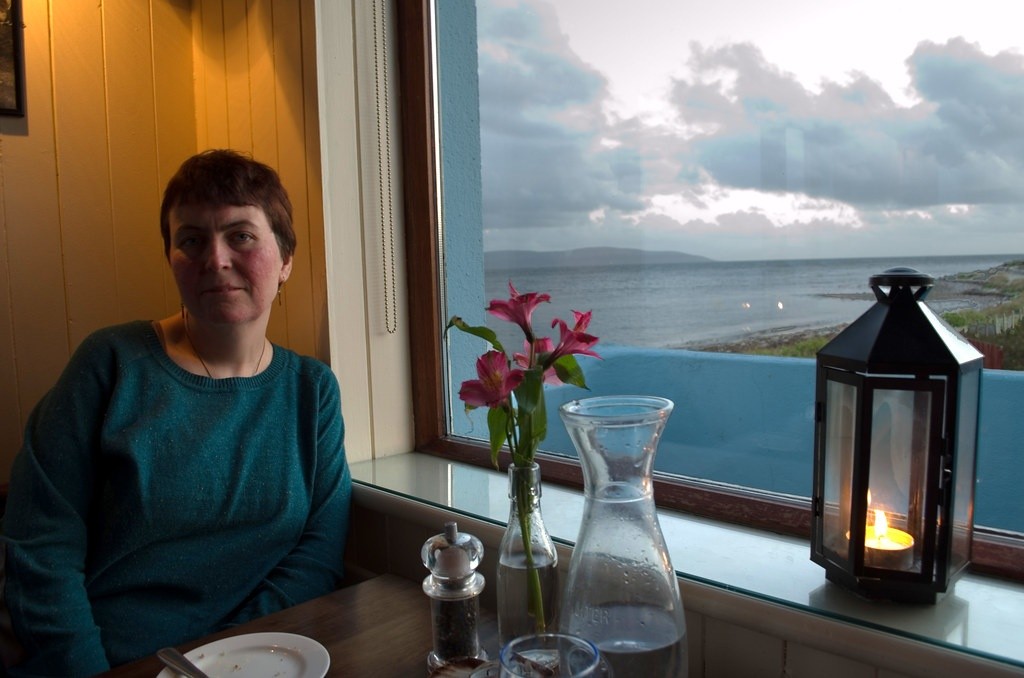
[181,306,266,383]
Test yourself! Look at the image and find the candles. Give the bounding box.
[848,512,914,568]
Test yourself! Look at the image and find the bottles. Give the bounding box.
[421,521,488,673]
[557,396,689,678]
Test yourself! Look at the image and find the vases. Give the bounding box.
[487,459,564,648]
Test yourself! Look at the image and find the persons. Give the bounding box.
[0,151,354,678]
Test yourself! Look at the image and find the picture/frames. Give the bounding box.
[0,0,24,116]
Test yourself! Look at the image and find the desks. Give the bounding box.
[104,563,499,678]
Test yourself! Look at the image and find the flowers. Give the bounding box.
[441,283,599,461]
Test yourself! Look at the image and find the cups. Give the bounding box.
[499,632,614,678]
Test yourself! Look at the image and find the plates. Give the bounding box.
[156,632,331,678]
